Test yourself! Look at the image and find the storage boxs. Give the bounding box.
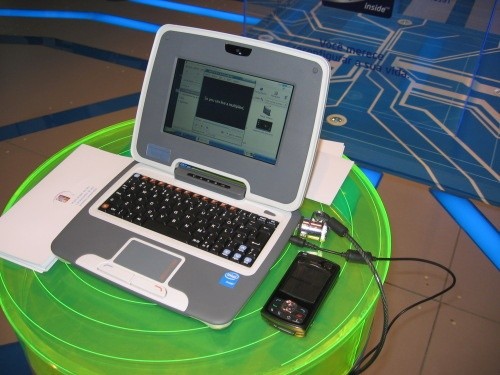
[0,119,394,375]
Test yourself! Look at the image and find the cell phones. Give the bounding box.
[261,251,341,336]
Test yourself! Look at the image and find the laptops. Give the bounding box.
[51,24,332,328]
